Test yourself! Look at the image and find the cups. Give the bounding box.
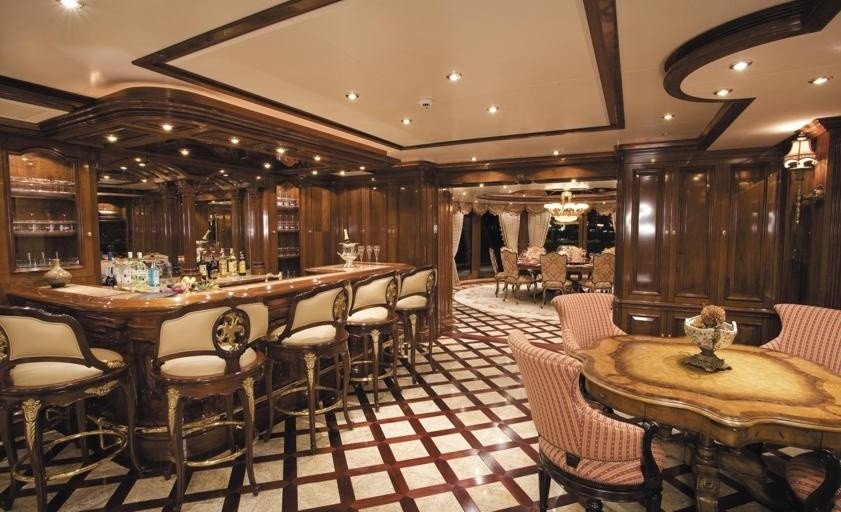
[10,176,80,264]
[277,189,299,256]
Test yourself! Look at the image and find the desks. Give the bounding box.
[569,333,841,511]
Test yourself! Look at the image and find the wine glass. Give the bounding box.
[339,244,359,268]
[359,245,382,264]
[683,314,739,373]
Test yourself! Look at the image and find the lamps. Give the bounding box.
[543,190,589,224]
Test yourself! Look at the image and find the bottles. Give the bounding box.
[209,213,216,240]
[343,228,352,254]
[43,259,73,287]
[200,247,247,280]
[104,251,160,287]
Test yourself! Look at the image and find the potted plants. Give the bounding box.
[679,302,741,374]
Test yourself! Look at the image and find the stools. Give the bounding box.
[1,306,147,511]
[262,280,355,452]
[333,264,438,414]
[149,297,269,511]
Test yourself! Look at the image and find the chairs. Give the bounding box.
[754,302,840,377]
[551,289,638,406]
[784,129,825,228]
[489,244,616,310]
[508,328,667,511]
[788,446,841,509]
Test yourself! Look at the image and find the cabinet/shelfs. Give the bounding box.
[303,164,440,343]
[0,113,303,281]
[613,140,788,346]
